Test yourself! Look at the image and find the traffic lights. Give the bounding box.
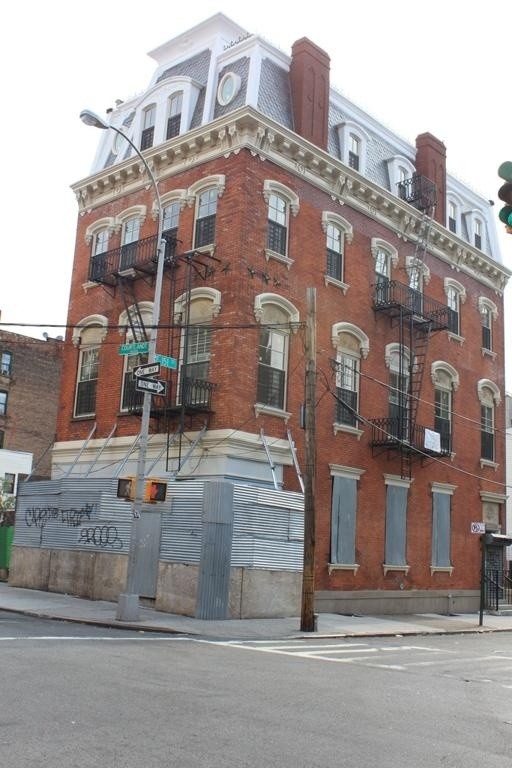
[497,160,512,233]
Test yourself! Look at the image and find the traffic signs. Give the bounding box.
[154,353,177,370]
[130,362,160,377]
[134,376,168,397]
[118,342,149,355]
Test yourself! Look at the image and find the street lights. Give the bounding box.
[75,105,169,622]
